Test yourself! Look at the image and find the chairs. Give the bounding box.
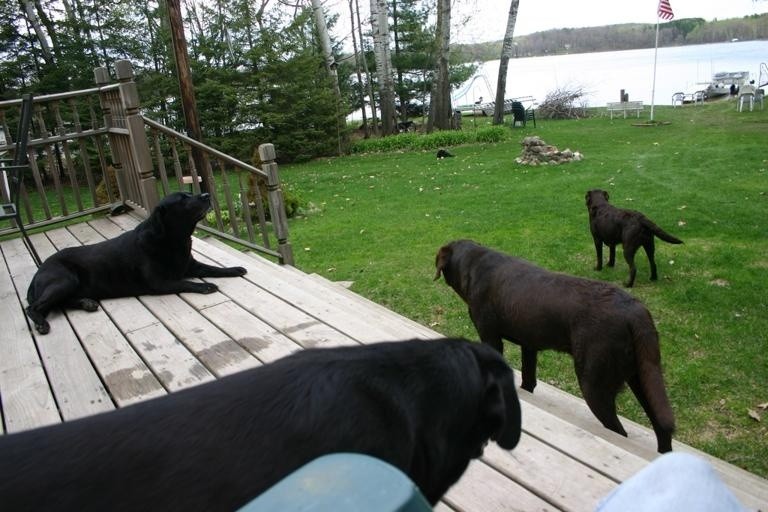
[672,92,685,109]
[0,93,42,266]
[512,102,537,128]
[737,85,756,112]
[694,90,705,107]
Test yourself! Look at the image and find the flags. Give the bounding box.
[656,0,674,21]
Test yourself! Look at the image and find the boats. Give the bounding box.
[680,67,755,104]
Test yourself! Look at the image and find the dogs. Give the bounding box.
[433,238,684,455]
[437,150,456,160]
[585,189,685,287]
[395,121,415,133]
[511,102,526,128]
[27,193,246,335]
[0,338,520,512]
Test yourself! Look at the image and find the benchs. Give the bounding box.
[606,101,644,119]
